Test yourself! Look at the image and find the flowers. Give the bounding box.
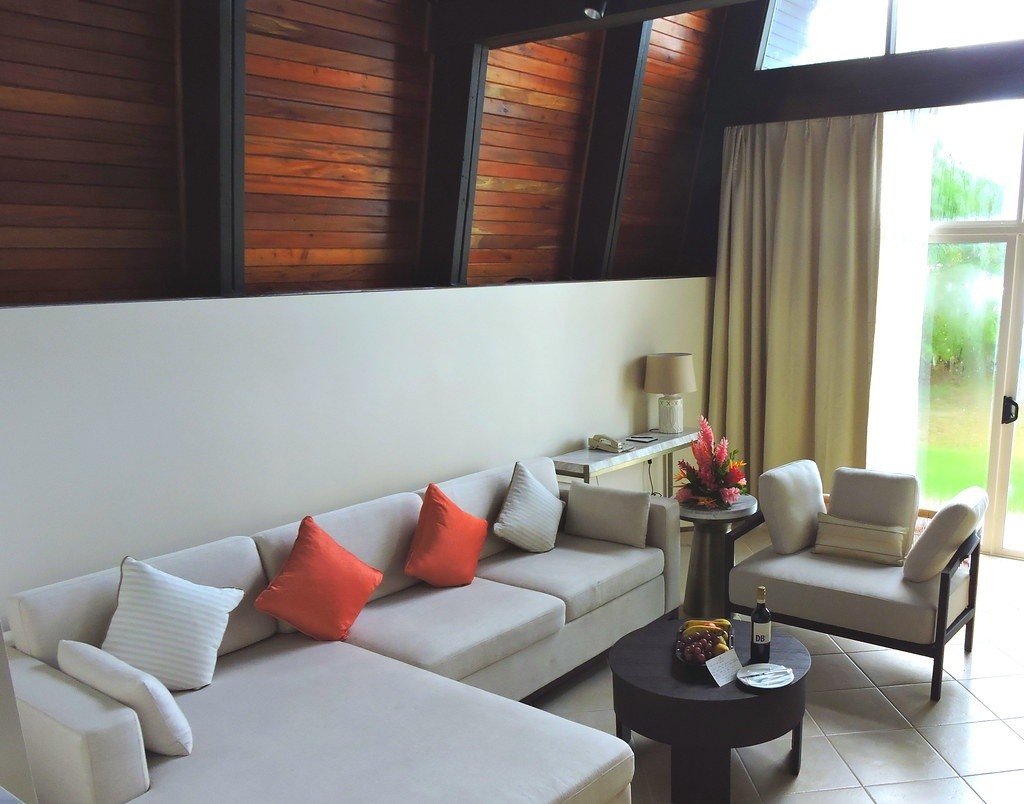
[670,416,749,511]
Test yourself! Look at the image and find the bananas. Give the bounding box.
[680,620,731,639]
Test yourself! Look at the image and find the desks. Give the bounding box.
[670,492,758,619]
[550,426,705,532]
[608,619,811,804]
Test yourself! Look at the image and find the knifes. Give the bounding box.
[739,668,793,679]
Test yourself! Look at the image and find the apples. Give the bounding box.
[712,618,729,657]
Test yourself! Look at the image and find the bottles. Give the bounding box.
[750,586,772,662]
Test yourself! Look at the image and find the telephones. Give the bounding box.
[588,434,635,453]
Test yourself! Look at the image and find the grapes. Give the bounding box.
[680,629,720,663]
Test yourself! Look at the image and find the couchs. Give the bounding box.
[726,493,982,702]
[1,456,682,804]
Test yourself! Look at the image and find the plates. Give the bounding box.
[737,663,794,689]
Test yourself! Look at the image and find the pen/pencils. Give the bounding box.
[631,436,652,438]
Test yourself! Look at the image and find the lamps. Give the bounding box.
[645,353,697,434]
[582,0,609,21]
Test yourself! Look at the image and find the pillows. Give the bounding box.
[825,467,920,557]
[100,556,245,691]
[404,483,489,588]
[493,461,566,553]
[58,640,193,756]
[563,480,651,549]
[252,516,384,641]
[810,512,911,567]
[902,486,989,583]
[759,459,828,555]
